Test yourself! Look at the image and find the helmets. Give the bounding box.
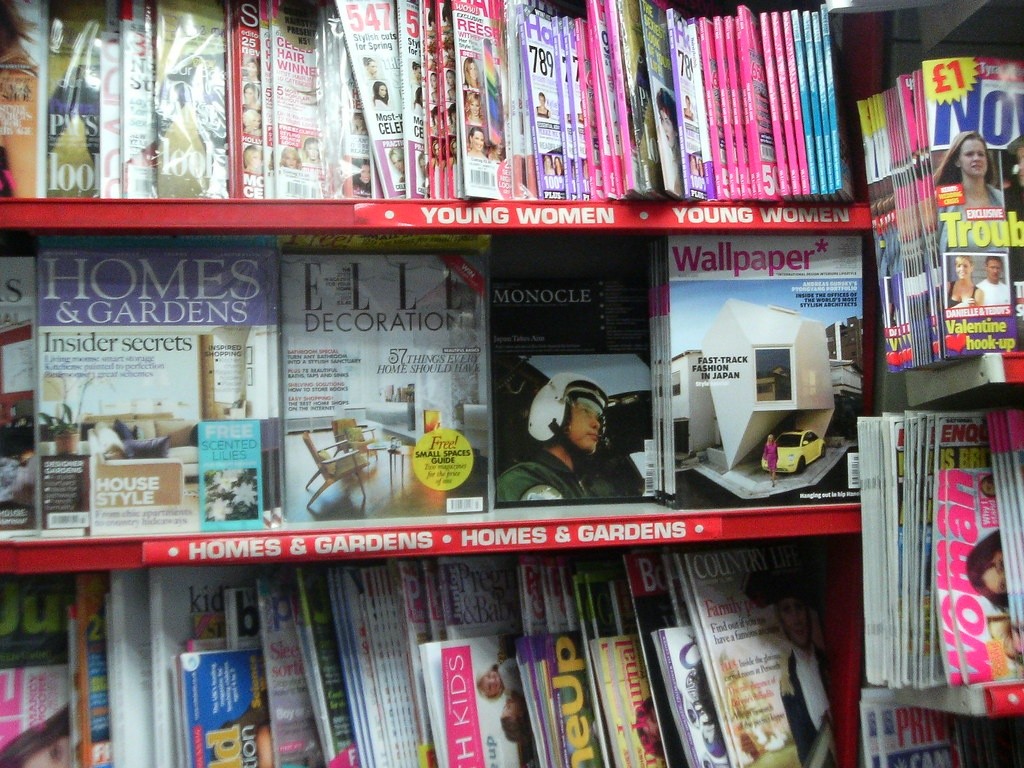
[527,373,609,443]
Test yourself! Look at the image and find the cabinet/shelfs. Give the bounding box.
[0,0,876,768]
[819,0,1024,768]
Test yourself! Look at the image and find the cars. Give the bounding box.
[761,428,827,475]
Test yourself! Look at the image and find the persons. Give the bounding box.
[946,255,985,308]
[682,96,694,121]
[976,256,1009,306]
[304,137,321,163]
[496,372,618,499]
[244,84,259,105]
[475,658,524,701]
[370,81,390,110]
[362,57,378,80]
[554,157,564,176]
[534,92,551,118]
[243,58,260,83]
[698,159,704,177]
[462,58,483,90]
[763,434,779,488]
[0,701,71,768]
[467,127,488,159]
[773,587,836,768]
[279,147,303,170]
[388,148,405,183]
[657,88,685,197]
[690,156,699,176]
[242,110,262,136]
[931,130,1007,253]
[243,146,262,174]
[465,92,487,126]
[543,156,555,175]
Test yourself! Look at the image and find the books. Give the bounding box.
[856,407,1024,691]
[0,0,867,768]
[854,55,1024,374]
